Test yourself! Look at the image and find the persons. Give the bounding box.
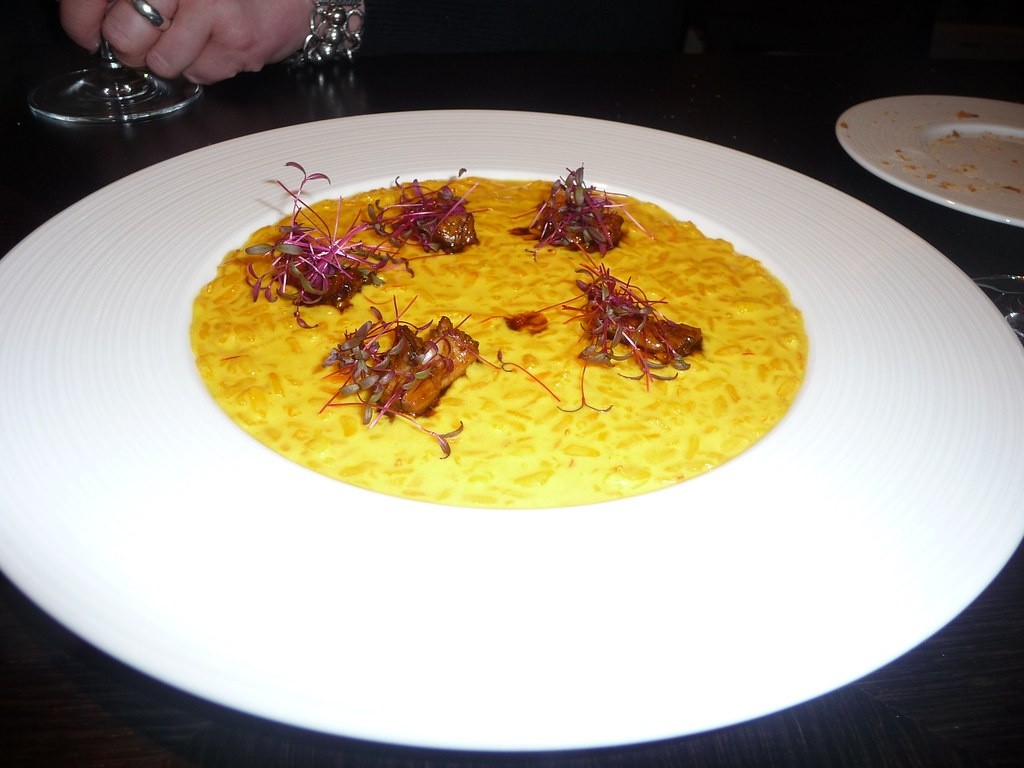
[57,0,936,87]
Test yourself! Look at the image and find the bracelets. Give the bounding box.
[286,0,366,63]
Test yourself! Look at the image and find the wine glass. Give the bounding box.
[24,37,204,125]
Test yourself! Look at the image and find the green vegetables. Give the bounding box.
[241,160,693,462]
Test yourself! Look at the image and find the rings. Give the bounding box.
[126,0,172,31]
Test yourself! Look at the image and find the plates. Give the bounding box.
[0,109,1024,752]
[833,95,1024,228]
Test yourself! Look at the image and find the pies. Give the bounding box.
[188,175,810,511]
